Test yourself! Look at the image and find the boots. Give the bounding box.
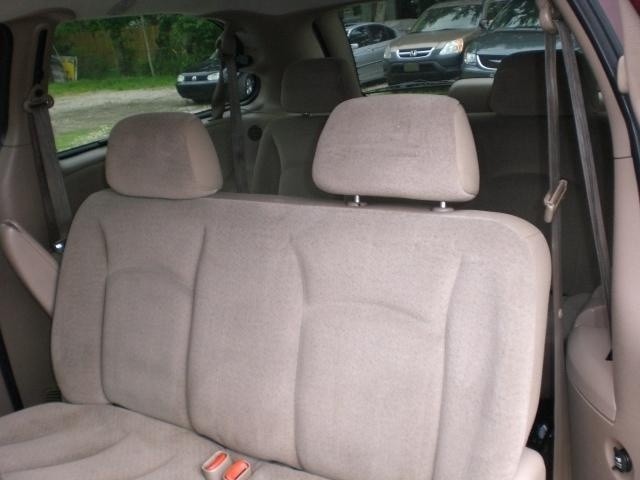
[383,1,504,90]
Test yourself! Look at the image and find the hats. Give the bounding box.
[459,0,584,79]
[174,45,256,105]
[343,21,402,85]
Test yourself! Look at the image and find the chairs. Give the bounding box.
[0,50,615,479]
